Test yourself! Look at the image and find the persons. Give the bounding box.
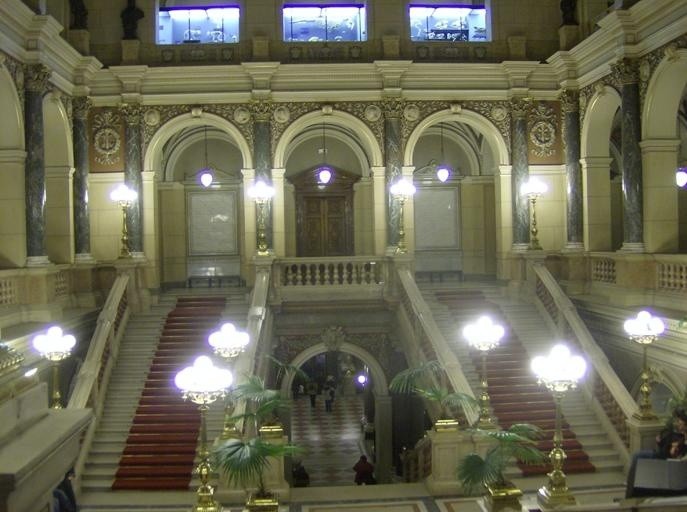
[292,370,337,413]
[288,456,311,489]
[624,407,687,501]
[352,455,377,485]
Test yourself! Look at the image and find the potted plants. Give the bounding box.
[205,399,307,512]
[457,422,551,512]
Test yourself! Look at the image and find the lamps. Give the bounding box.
[176,322,252,512]
[436,121,451,183]
[319,123,331,184]
[109,184,138,256]
[462,314,505,429]
[199,125,213,187]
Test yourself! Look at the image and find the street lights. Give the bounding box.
[110,183,140,261]
[209,319,251,447]
[386,178,416,257]
[172,351,235,512]
[244,180,278,260]
[457,315,509,430]
